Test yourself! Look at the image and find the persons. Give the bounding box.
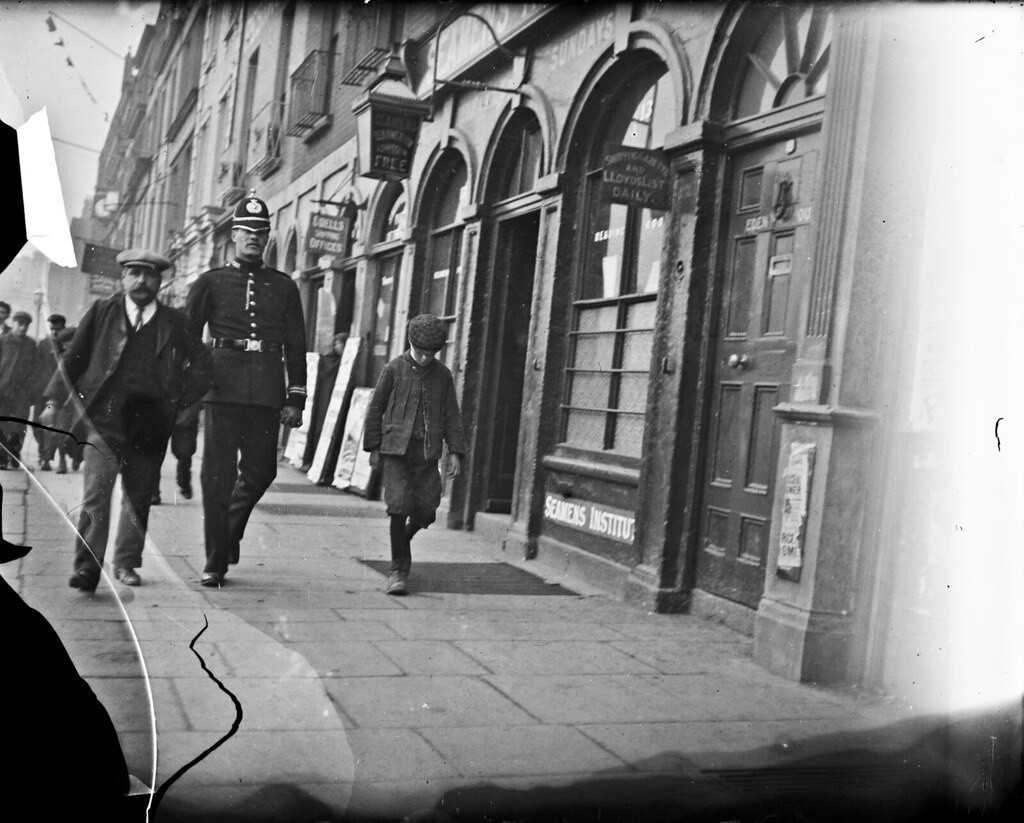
[0,195,307,589]
[362,314,467,593]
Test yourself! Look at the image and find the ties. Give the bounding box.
[133,306,146,333]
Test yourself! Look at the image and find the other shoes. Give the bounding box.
[385,571,408,594]
[72,458,80,471]
[151,491,161,505]
[10,452,20,467]
[201,570,225,585]
[42,462,51,471]
[56,466,66,473]
[228,541,240,564]
[113,565,140,585]
[69,570,96,592]
[181,484,193,499]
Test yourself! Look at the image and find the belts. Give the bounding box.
[212,337,280,352]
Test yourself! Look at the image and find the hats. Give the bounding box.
[48,315,65,323]
[230,198,271,232]
[115,249,170,273]
[12,312,32,322]
[0,486,32,566]
[57,327,77,342]
[409,313,448,351]
